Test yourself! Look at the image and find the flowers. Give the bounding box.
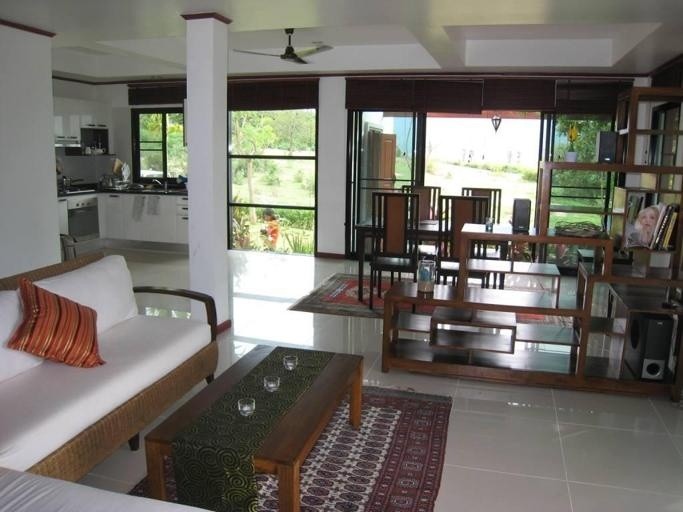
[567,124,578,152]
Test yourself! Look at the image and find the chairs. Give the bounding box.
[367,185,508,314]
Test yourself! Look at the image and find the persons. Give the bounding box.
[260,209,279,252]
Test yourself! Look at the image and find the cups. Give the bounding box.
[237,354,299,415]
[484,217,495,234]
[417,260,437,295]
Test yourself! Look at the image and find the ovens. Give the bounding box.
[58,195,97,235]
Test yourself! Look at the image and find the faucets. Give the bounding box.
[152,178,162,185]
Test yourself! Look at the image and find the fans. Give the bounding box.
[233,28,333,64]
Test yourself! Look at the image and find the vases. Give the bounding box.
[565,151,578,162]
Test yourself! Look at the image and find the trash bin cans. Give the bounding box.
[59,233,80,263]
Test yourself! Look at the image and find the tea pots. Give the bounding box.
[99,175,112,191]
[82,145,105,155]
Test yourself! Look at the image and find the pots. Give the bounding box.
[61,175,81,186]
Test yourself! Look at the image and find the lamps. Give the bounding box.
[491,79,501,131]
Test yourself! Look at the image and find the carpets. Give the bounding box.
[126,384,453,512]
[287,271,573,330]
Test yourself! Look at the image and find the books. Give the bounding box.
[626,194,679,251]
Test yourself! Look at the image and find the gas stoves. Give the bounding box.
[57,183,97,195]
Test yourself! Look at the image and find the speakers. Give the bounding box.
[622,310,674,381]
[513,199,530,230]
[596,131,617,163]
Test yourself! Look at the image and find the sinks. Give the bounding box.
[141,189,176,193]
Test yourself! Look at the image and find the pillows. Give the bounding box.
[16,253,139,361]
[7,278,106,368]
[0,289,43,384]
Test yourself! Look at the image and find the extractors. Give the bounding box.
[54,136,79,157]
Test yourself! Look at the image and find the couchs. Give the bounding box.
[1,246,219,483]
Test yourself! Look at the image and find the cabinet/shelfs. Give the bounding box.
[80,127,109,154]
[98,194,188,244]
[610,185,656,237]
[80,100,107,126]
[53,97,80,147]
[381,85,683,403]
